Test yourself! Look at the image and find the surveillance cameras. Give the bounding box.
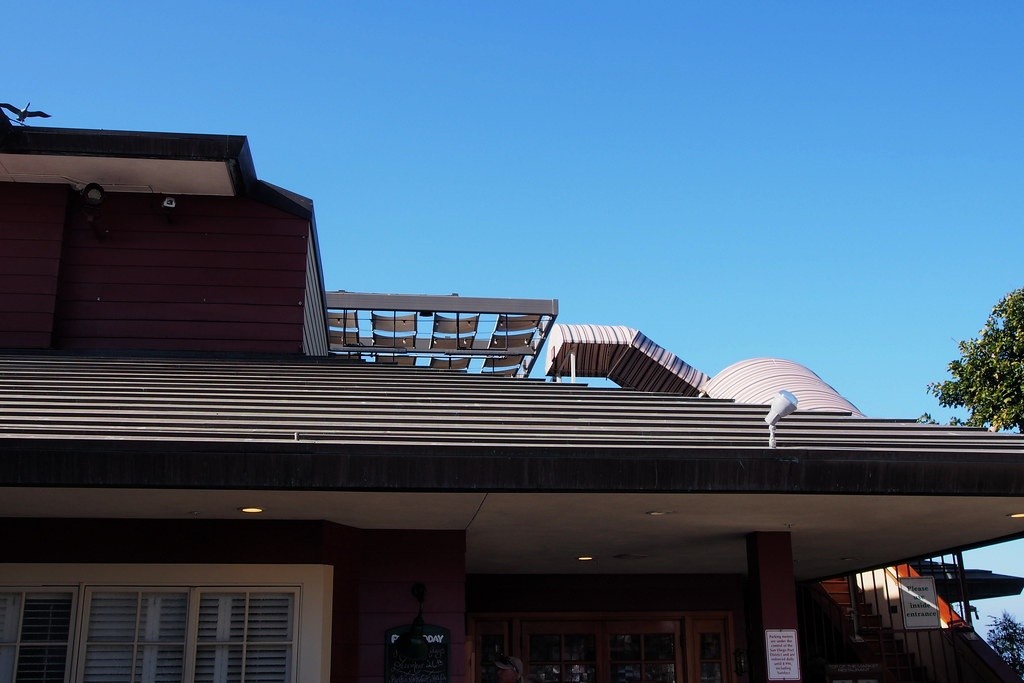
[162,198,176,208]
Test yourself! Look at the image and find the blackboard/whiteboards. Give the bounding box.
[385,624,451,683]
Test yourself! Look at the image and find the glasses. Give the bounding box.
[495,653,519,674]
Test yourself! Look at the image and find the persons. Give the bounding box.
[494,656,525,683]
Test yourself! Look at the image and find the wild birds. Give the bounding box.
[0,102,52,123]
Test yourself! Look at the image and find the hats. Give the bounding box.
[495,656,523,680]
